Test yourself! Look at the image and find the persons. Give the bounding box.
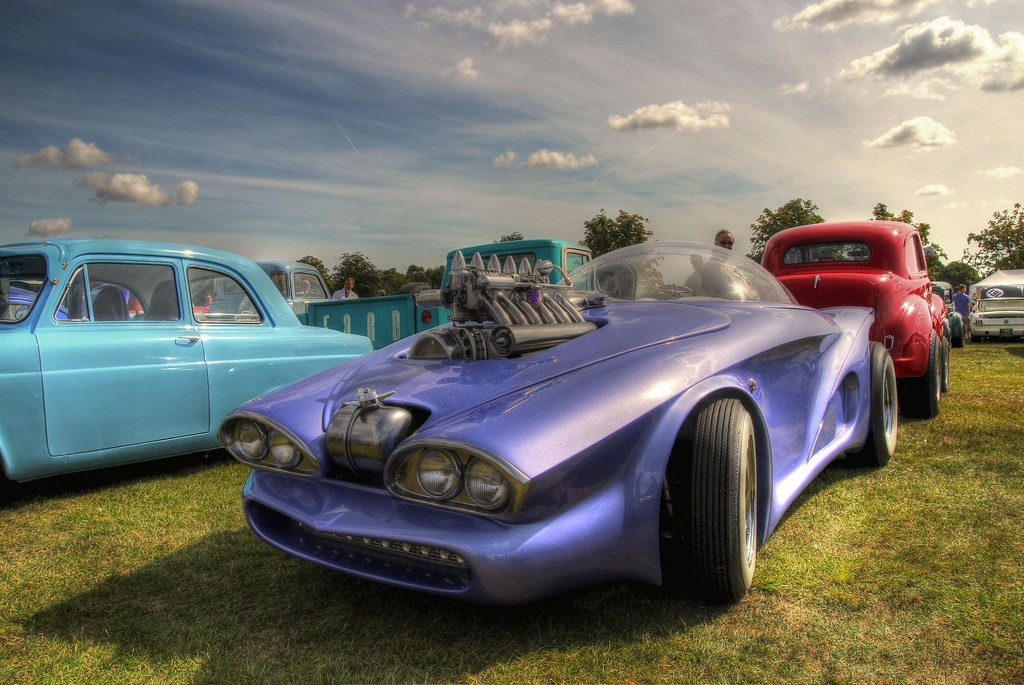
[701,230,762,303]
[203,294,212,307]
[296,280,316,295]
[953,284,972,343]
[332,277,358,299]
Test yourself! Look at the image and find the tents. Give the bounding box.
[969,269,1024,297]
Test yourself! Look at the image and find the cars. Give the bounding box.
[931,280,965,348]
[0,237,375,507]
[0,259,211,324]
[760,219,951,419]
[969,286,1024,342]
[204,260,339,322]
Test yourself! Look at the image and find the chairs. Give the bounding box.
[91,281,180,321]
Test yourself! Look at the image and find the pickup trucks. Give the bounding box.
[297,240,592,354]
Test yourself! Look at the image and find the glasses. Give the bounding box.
[718,240,733,245]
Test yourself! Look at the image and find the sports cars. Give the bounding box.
[218,239,899,608]
